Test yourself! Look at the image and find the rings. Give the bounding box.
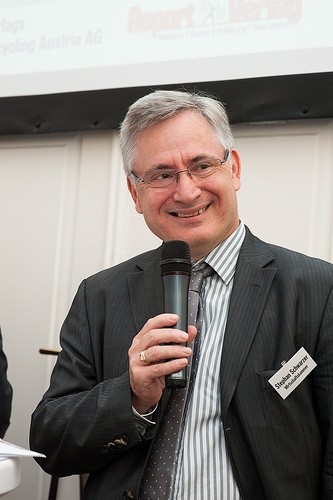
[139,350,151,364]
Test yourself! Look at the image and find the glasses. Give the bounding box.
[130,146,230,190]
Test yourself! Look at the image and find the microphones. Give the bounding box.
[160,240,192,389]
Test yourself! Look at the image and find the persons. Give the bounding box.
[28,88,333,499]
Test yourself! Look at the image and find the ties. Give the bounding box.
[138,264,216,500]
